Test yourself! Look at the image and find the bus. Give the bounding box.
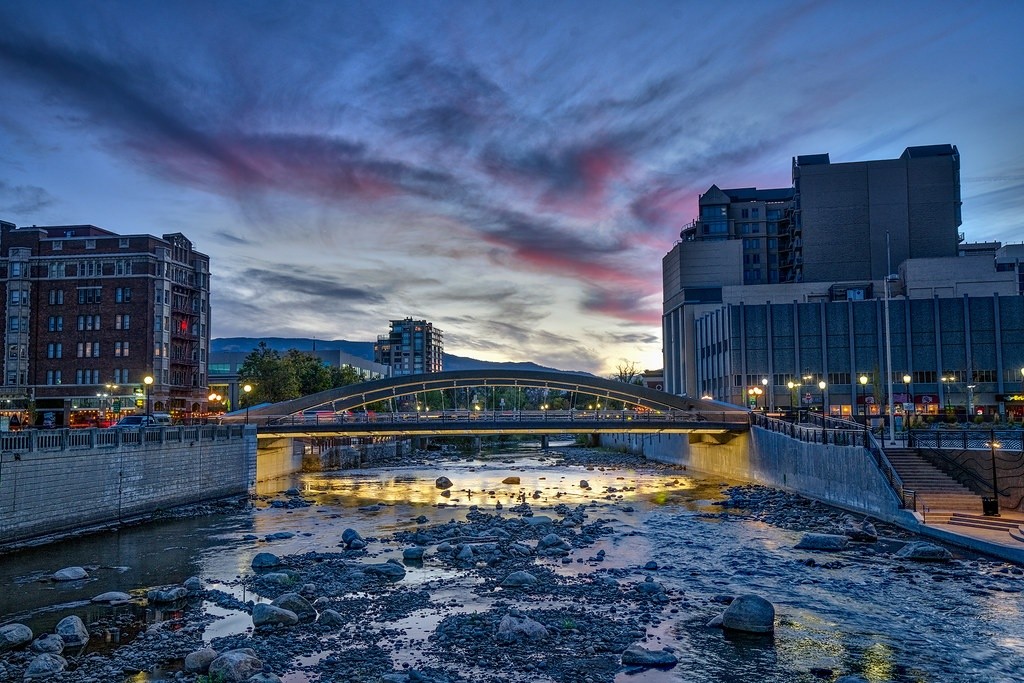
[418,415,441,422]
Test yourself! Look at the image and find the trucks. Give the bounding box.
[107,411,173,428]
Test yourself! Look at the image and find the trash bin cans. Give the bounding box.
[982,497,999,516]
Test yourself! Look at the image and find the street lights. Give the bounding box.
[860,375,869,429]
[967,385,977,415]
[818,381,826,429]
[941,377,955,414]
[749,387,763,409]
[803,375,813,411]
[105,376,118,426]
[794,382,801,411]
[701,395,713,400]
[762,379,768,410]
[1021,368,1024,419]
[903,374,912,429]
[244,385,252,425]
[788,381,794,414]
[143,376,154,427]
[96,389,109,423]
[208,393,222,416]
[416,404,601,413]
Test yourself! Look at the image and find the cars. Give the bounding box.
[689,416,708,422]
[43,420,57,429]
